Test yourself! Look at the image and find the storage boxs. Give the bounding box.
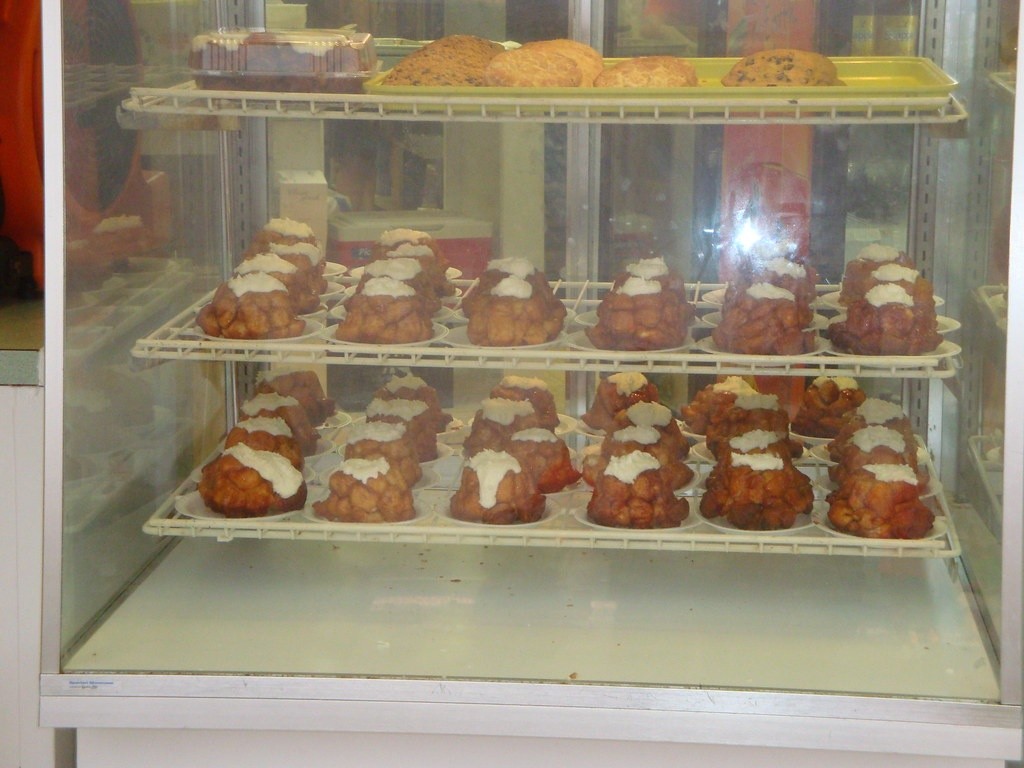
[272,169,328,253]
[328,208,493,311]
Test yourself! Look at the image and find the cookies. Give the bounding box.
[380,31,698,92]
[719,47,845,87]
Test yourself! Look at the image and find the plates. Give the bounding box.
[192,261,963,361]
[176,408,949,551]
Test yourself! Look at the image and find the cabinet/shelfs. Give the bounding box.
[117,53,968,561]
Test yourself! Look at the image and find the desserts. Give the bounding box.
[198,215,944,540]
[189,31,374,98]
